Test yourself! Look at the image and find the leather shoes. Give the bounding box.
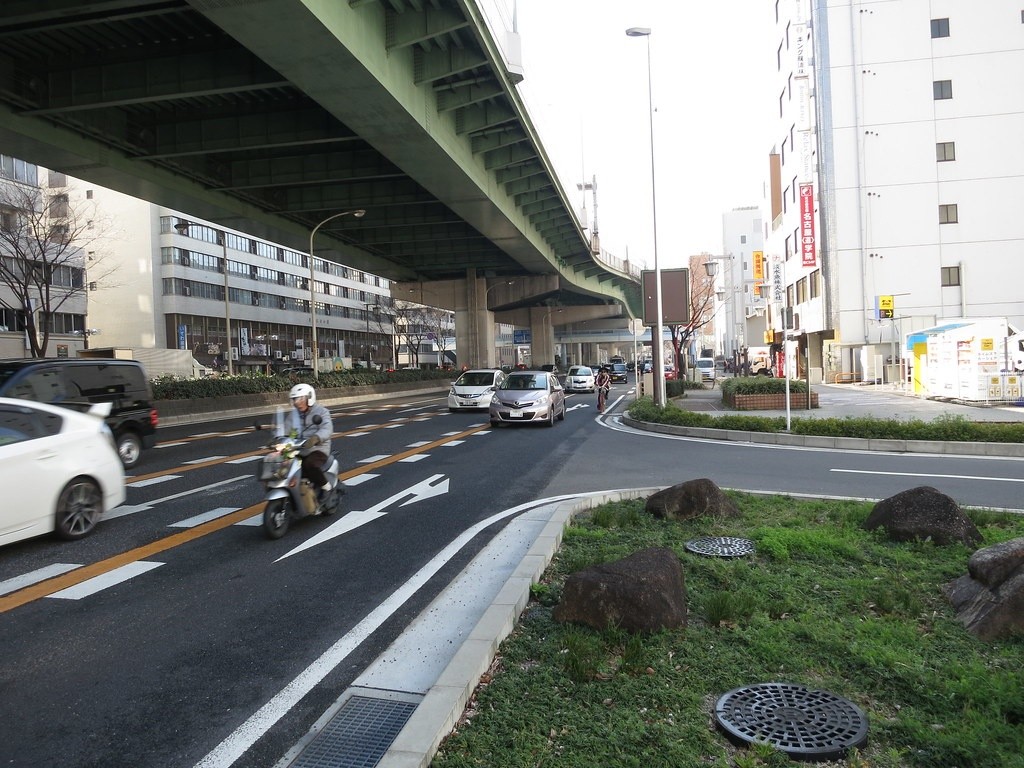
[317,486,333,502]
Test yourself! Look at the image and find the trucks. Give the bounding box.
[749,357,772,375]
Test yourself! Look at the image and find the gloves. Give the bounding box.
[302,435,319,449]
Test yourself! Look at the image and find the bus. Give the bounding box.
[610,355,625,364]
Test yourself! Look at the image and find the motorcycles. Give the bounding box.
[252,414,342,538]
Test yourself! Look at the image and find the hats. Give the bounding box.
[598,367,609,373]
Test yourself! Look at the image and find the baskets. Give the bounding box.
[257,458,293,481]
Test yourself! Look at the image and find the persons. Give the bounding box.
[595,369,610,410]
[474,376,480,382]
[274,384,334,503]
[724,359,728,373]
[519,378,524,388]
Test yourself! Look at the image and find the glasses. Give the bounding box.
[602,372,606,373]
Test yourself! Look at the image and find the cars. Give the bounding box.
[489,368,566,426]
[541,364,560,375]
[625,356,654,373]
[270,366,320,376]
[0,397,127,550]
[593,368,599,376]
[565,365,595,393]
[603,364,612,369]
[665,366,679,380]
[609,364,628,384]
[447,369,506,411]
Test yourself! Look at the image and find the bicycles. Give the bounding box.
[594,385,610,413]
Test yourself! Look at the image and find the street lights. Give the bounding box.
[310,208,366,379]
[409,287,442,368]
[486,280,516,311]
[74,328,98,349]
[542,309,566,364]
[702,252,739,357]
[173,221,235,377]
[626,24,672,410]
[363,303,382,370]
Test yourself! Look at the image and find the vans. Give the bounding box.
[0,356,159,469]
[696,358,717,382]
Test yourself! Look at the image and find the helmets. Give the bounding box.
[289,383,316,406]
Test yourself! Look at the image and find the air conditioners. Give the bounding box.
[183,256,189,266]
[216,236,348,315]
[289,351,298,359]
[184,287,191,295]
[273,350,282,359]
[223,347,239,360]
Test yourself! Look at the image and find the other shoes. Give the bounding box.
[605,395,608,399]
[597,404,600,410]
